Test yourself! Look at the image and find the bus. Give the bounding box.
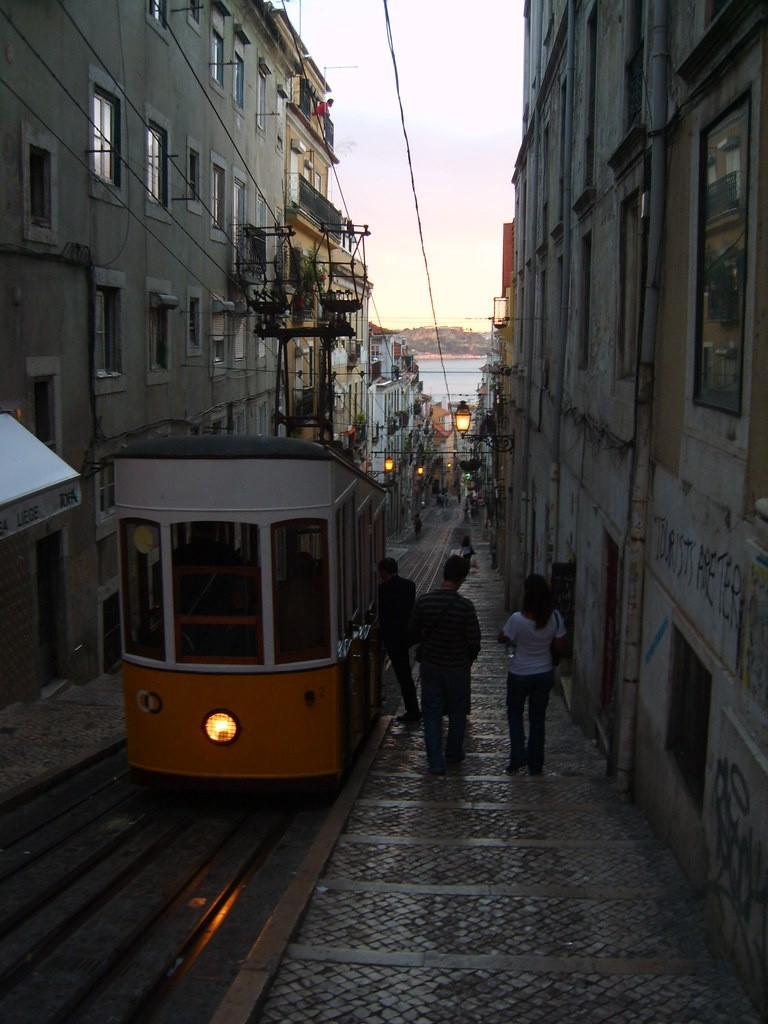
[105,432,391,797]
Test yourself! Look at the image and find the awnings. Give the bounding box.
[0,413,82,540]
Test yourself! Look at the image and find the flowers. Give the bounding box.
[320,269,327,282]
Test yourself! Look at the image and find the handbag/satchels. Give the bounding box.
[551,611,560,666]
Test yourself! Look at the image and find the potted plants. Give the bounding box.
[302,241,316,290]
[294,285,305,308]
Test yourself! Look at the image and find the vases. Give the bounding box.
[315,285,321,291]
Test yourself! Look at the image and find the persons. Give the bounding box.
[497,574,567,776]
[459,536,476,569]
[457,491,461,503]
[408,555,481,776]
[414,514,422,536]
[314,99,334,119]
[378,557,421,722]
[464,499,468,513]
[469,503,475,519]
[437,491,448,508]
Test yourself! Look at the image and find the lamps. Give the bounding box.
[455,401,515,453]
[367,457,394,478]
[418,467,423,475]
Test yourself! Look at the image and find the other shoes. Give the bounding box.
[429,757,446,774]
[510,751,527,769]
[396,711,421,723]
[445,748,465,763]
[528,760,543,776]
